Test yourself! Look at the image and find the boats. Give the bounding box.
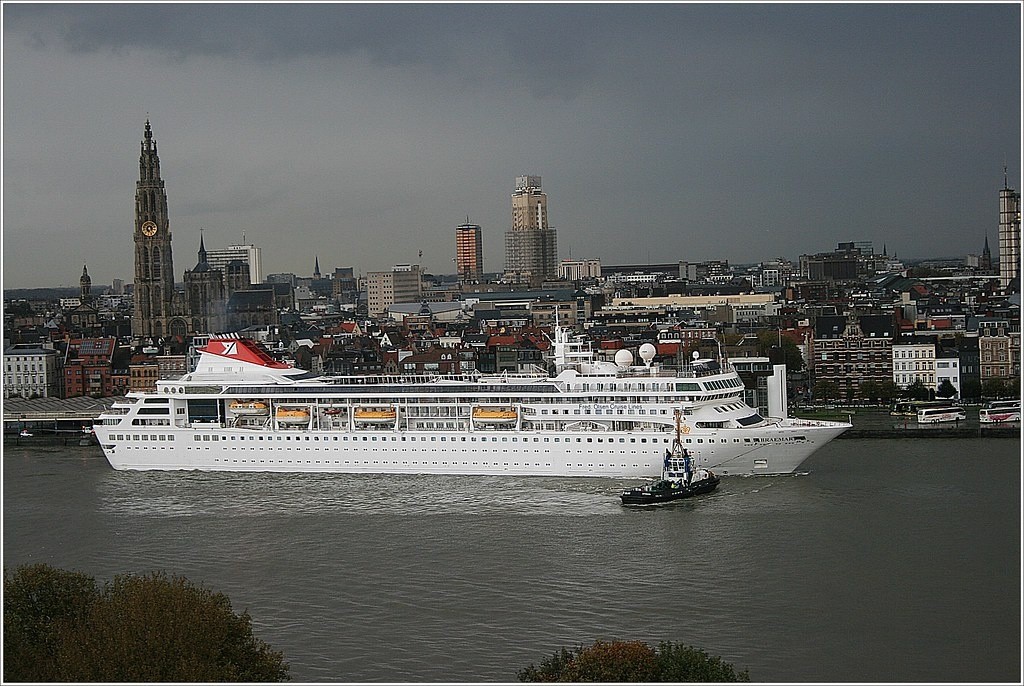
[324,410,343,414]
[473,411,517,424]
[275,410,309,425]
[621,404,720,508]
[355,413,396,423]
[230,403,265,415]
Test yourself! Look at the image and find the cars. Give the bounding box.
[20,430,33,438]
[78,426,93,435]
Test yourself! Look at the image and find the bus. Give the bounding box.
[918,406,968,424]
[979,407,1020,423]
[982,400,1020,409]
[890,402,958,415]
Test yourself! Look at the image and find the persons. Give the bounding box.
[670,481,675,489]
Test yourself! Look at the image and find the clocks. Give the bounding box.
[141,221,158,236]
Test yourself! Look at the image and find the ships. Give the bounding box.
[92,338,853,478]
